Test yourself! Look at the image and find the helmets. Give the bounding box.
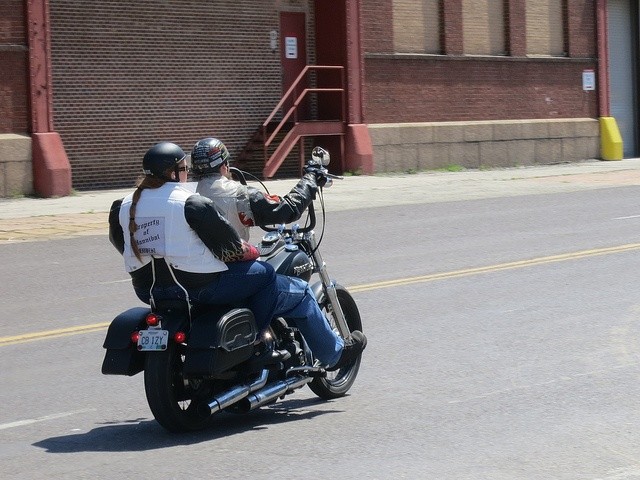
[191,138,229,174]
[143,143,186,182]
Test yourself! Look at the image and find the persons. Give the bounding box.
[190,137,367,371]
[108,140,277,353]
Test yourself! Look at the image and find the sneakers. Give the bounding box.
[325,330,367,369]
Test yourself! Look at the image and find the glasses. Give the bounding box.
[178,165,189,173]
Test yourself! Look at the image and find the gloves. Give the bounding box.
[306,168,327,186]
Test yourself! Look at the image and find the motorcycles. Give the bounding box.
[100,146,363,431]
